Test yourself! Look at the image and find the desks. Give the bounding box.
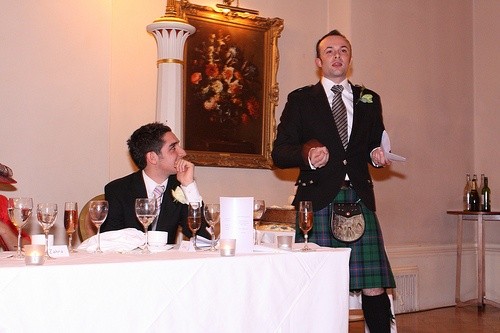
[0,241,352,333]
[447,211,500,313]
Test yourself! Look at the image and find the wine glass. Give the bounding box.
[135,197,157,251]
[254,200,266,249]
[187,203,200,250]
[203,203,221,251]
[36,202,57,260]
[298,201,313,250]
[8,198,33,260]
[89,200,109,255]
[64,201,78,253]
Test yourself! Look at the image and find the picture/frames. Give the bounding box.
[180,3,284,171]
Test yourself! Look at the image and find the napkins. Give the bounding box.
[79,226,147,253]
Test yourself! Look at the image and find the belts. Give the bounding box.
[338,180,354,190]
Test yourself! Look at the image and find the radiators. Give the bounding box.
[392,267,418,314]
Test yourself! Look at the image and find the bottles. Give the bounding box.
[464,174,491,212]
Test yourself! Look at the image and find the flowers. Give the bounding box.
[172,183,189,206]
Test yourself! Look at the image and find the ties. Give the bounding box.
[330,85,349,152]
[150,185,166,231]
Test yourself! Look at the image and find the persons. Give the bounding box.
[100,120,212,245]
[0,163,32,251]
[272,29,397,333]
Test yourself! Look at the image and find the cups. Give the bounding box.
[30,234,54,249]
[146,230,168,249]
[278,236,292,250]
[219,238,236,256]
[23,243,45,265]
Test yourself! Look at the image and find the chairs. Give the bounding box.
[79,195,108,241]
